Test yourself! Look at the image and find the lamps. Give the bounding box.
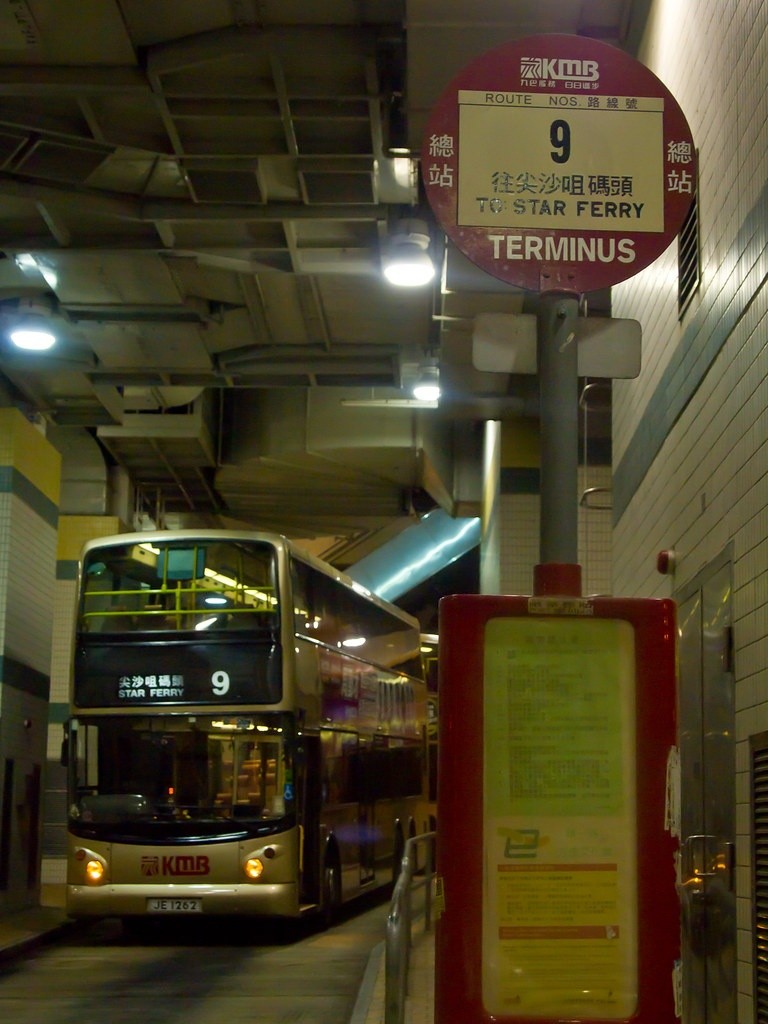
[381,216,436,289]
[10,294,55,350]
[412,366,442,401]
[409,485,440,522]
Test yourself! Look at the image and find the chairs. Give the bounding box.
[199,755,281,808]
[98,602,274,630]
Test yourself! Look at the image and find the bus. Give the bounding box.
[60,527,429,920]
